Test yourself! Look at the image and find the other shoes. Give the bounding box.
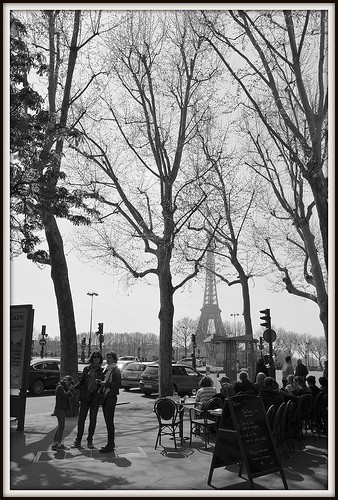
[52,444,57,449]
[57,444,70,450]
[100,449,114,453]
[101,443,115,448]
[71,440,81,448]
[87,438,94,448]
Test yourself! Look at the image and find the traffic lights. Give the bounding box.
[98,322,103,334]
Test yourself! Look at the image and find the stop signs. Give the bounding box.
[83,343,86,348]
[39,339,47,346]
[193,343,197,348]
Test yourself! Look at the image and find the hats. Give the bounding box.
[218,376,230,383]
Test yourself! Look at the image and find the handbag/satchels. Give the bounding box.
[92,392,106,407]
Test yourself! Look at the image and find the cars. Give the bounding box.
[102,361,135,377]
[27,360,83,394]
[177,357,203,367]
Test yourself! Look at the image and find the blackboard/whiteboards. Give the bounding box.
[210,394,281,479]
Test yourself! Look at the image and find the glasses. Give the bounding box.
[93,355,100,358]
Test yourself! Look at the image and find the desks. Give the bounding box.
[174,400,197,439]
[208,409,253,417]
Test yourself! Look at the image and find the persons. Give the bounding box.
[94,351,122,453]
[51,376,75,451]
[70,351,104,448]
[192,355,328,436]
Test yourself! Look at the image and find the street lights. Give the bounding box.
[87,290,98,357]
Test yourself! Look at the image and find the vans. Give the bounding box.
[117,355,139,362]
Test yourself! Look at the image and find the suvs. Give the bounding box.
[139,364,205,396]
[120,361,159,392]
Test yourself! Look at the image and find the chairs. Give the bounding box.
[152,389,328,459]
